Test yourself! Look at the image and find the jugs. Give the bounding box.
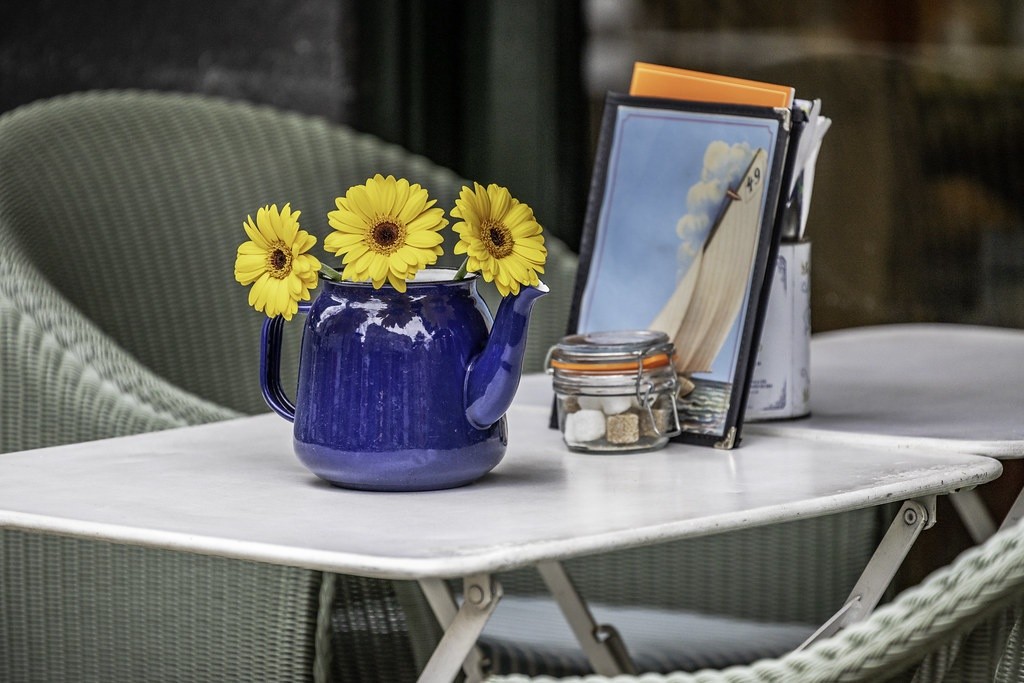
[258,265,549,490]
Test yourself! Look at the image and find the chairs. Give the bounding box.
[0,90,879,683]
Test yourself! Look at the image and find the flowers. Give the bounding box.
[237,174,548,322]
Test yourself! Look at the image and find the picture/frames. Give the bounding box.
[539,89,806,454]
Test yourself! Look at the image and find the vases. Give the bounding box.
[260,266,551,494]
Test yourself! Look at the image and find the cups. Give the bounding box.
[744,239,813,423]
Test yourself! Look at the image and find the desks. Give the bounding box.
[1,323,1023,682]
